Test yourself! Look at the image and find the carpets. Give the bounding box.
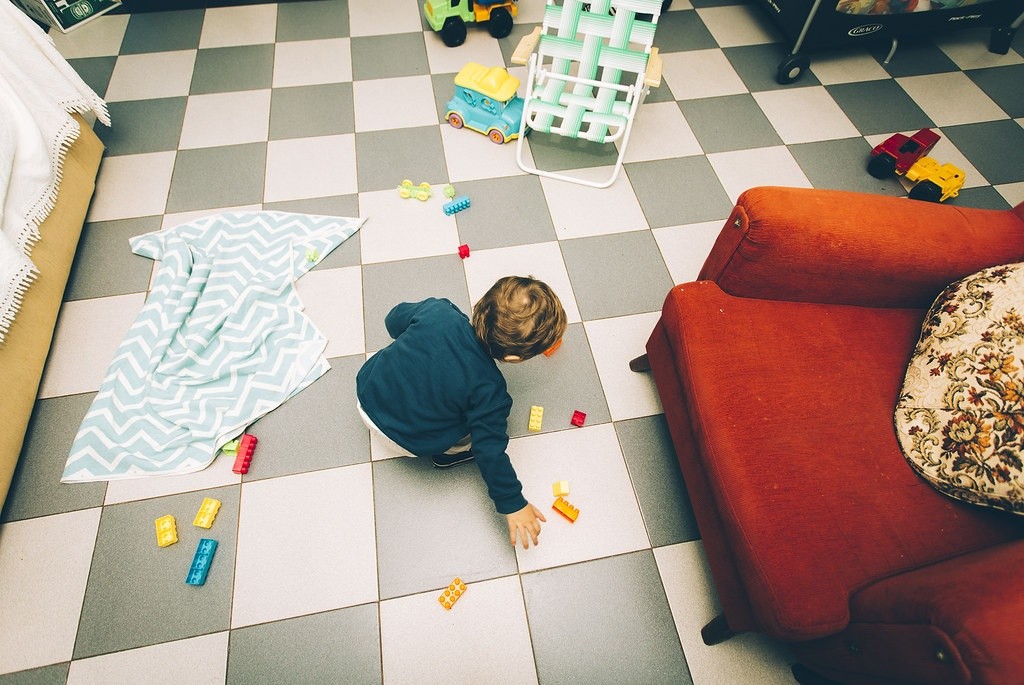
[60,208,367,485]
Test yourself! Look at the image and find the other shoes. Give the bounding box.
[431,448,477,470]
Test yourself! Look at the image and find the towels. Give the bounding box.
[58,208,369,484]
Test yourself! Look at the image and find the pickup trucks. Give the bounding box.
[865,127,968,203]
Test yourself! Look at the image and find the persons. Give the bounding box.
[356,277,566,549]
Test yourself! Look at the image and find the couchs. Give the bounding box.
[0,0,112,517]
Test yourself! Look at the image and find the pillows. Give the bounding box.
[893,259,1024,515]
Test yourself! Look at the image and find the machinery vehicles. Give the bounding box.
[422,0,519,47]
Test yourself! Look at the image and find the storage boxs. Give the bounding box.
[10,0,123,33]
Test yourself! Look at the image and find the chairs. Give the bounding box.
[516,0,664,188]
[628,184,1024,684]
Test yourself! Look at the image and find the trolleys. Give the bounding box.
[582,0,1024,85]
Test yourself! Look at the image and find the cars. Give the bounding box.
[443,60,535,144]
